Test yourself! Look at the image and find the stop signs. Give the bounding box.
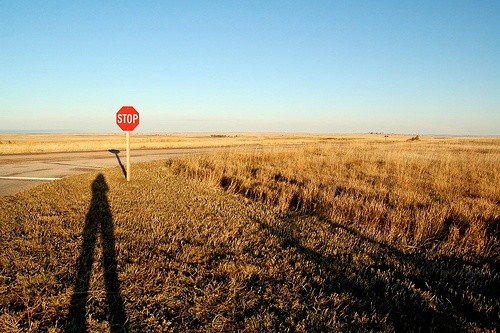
[116,106,139,131]
